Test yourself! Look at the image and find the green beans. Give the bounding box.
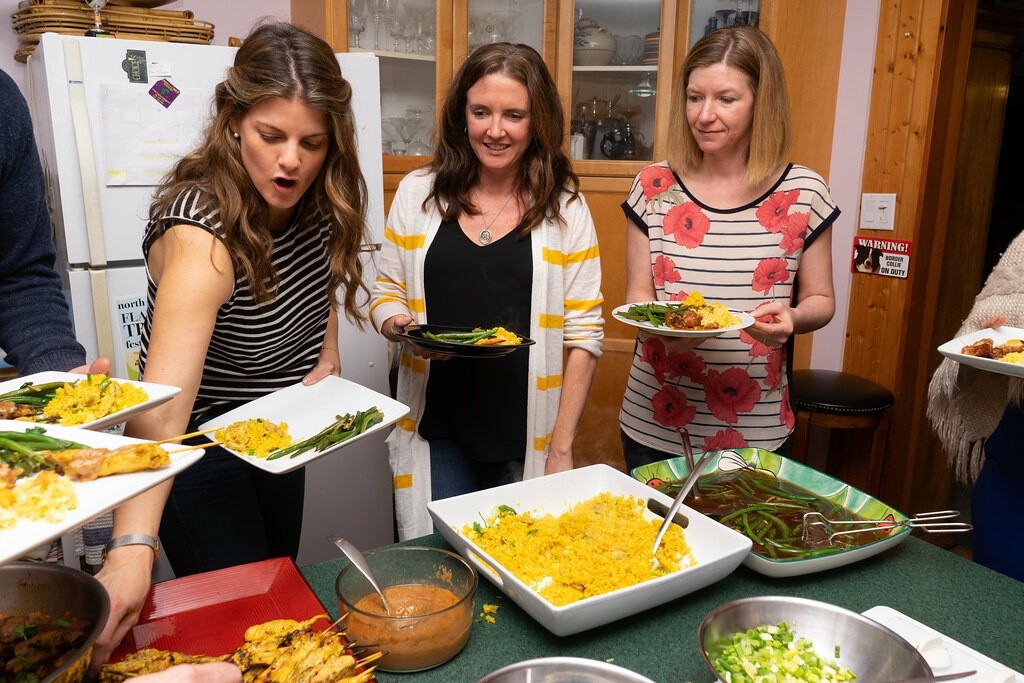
[0,426,91,468]
[267,407,384,460]
[617,302,688,328]
[659,472,885,557]
[0,382,73,405]
[422,330,498,344]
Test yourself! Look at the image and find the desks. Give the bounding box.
[303,529,1024,683]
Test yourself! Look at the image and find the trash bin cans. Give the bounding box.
[788,369,894,501]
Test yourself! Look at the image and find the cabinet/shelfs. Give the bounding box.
[291,0,849,470]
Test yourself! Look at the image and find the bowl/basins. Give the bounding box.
[335,547,477,673]
[698,595,937,683]
[612,34,645,66]
[476,656,656,683]
[1,562,114,683]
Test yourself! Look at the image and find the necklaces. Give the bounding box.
[472,179,522,245]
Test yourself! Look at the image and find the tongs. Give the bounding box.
[803,509,973,548]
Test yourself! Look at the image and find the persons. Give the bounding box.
[621,24,841,478]
[0,68,112,375]
[370,42,605,544]
[87,24,367,678]
[927,230,1024,583]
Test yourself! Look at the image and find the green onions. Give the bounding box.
[716,622,856,683]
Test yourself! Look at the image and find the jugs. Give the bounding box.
[571,99,649,162]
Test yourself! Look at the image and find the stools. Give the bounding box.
[788,367,895,498]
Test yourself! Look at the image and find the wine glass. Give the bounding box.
[347,1,521,160]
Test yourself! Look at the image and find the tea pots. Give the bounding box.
[572,18,618,67]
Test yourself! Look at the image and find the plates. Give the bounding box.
[110,555,377,683]
[425,462,752,637]
[393,325,534,357]
[630,446,912,581]
[611,300,757,338]
[938,327,1023,378]
[0,372,181,434]
[0,419,207,572]
[197,372,412,475]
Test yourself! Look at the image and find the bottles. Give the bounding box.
[704,0,760,36]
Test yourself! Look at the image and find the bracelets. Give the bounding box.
[102,533,160,568]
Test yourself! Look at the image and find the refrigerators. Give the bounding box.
[24,31,397,585]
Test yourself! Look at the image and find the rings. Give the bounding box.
[763,340,766,344]
